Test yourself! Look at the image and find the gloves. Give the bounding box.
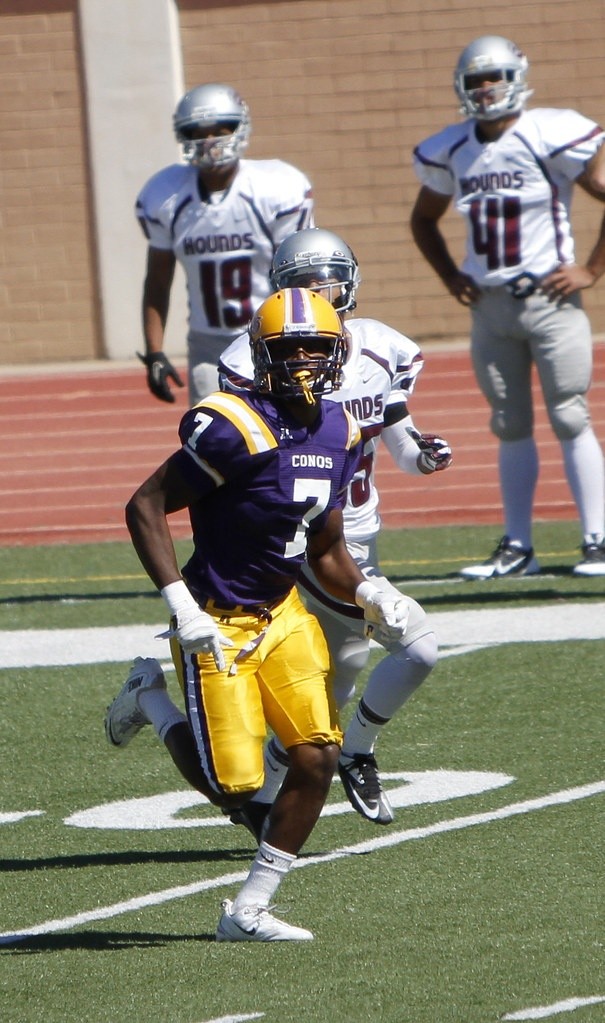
[408,426,452,472]
[161,580,233,672]
[135,349,185,404]
[353,581,409,642]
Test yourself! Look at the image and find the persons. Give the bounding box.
[134,85,315,413]
[220,229,453,850]
[102,290,406,942]
[407,37,605,578]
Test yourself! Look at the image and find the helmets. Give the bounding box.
[172,82,250,171]
[249,286,350,395]
[454,37,533,120]
[272,227,360,309]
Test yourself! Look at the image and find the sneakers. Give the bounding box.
[574,534,605,576]
[216,898,315,942]
[459,533,538,578]
[104,656,168,749]
[222,797,274,844]
[338,749,394,827]
